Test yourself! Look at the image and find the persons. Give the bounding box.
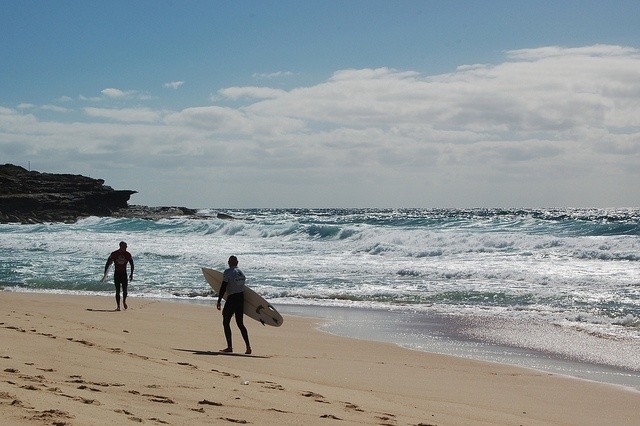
[217,255,251,354]
[105,241,134,311]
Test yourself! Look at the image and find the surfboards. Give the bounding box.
[99,261,114,284]
[201,266,283,327]
